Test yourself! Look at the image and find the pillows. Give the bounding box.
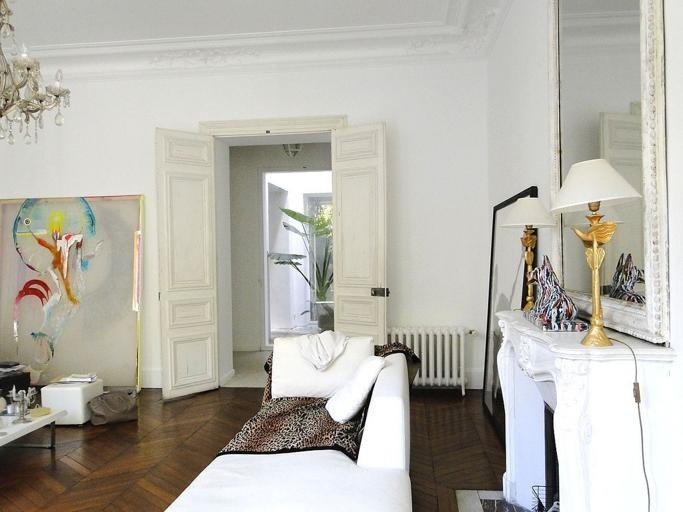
[271,335,388,424]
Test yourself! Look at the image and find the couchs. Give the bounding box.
[161,333,414,511]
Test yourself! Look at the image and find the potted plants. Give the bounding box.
[266,204,335,330]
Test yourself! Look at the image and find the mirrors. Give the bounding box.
[547,0,669,344]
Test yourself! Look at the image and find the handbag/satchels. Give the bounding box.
[86,389,138,426]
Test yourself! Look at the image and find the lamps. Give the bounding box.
[0,1,70,147]
[548,157,645,345]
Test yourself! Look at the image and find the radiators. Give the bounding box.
[386,323,476,397]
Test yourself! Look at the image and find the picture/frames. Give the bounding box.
[480,186,540,432]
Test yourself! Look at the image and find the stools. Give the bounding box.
[39,379,104,428]
